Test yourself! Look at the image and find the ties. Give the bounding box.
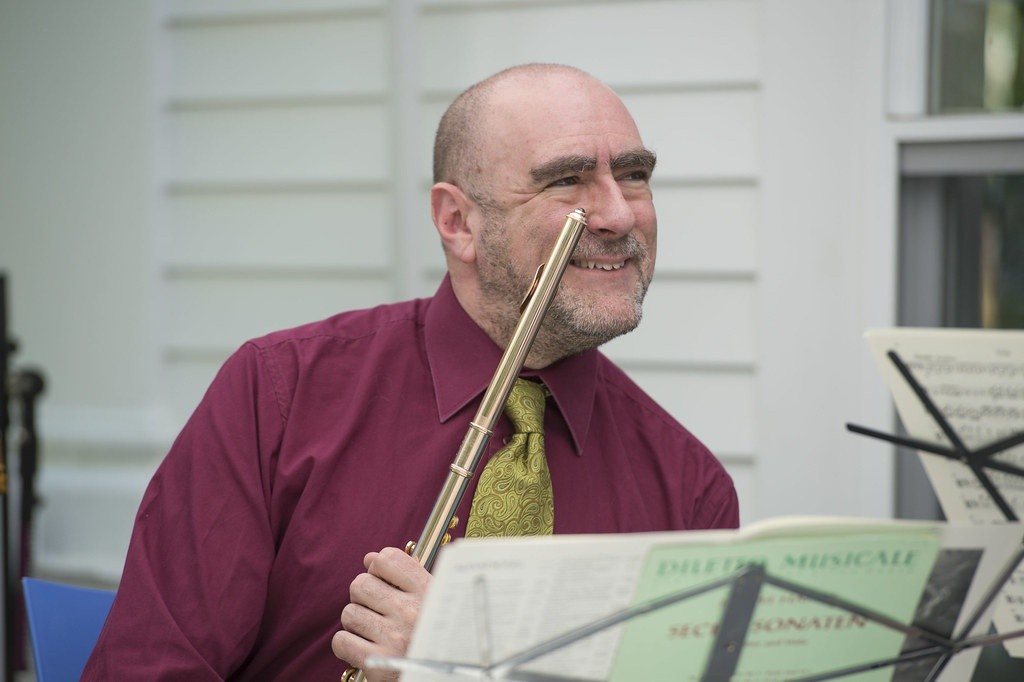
[464,380,556,537]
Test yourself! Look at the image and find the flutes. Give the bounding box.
[339,207,589,682]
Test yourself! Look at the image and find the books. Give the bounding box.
[862,326,1024,657]
[403,516,1024,682]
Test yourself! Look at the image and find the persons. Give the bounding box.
[76,63,741,682]
